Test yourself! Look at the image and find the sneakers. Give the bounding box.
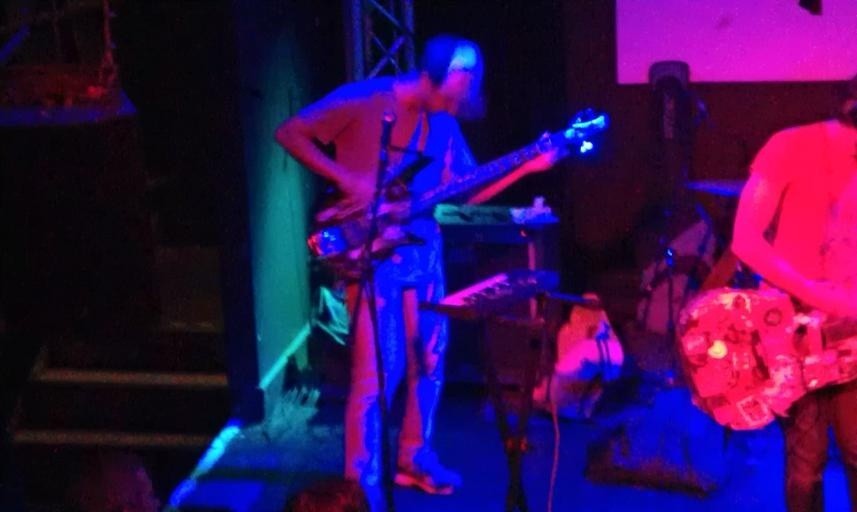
[395,464,452,496]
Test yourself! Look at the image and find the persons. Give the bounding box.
[270,35,562,497]
[723,74,854,511]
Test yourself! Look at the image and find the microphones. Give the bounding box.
[380,111,397,148]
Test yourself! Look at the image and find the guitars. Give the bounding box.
[309,109,611,283]
[678,288,856,430]
